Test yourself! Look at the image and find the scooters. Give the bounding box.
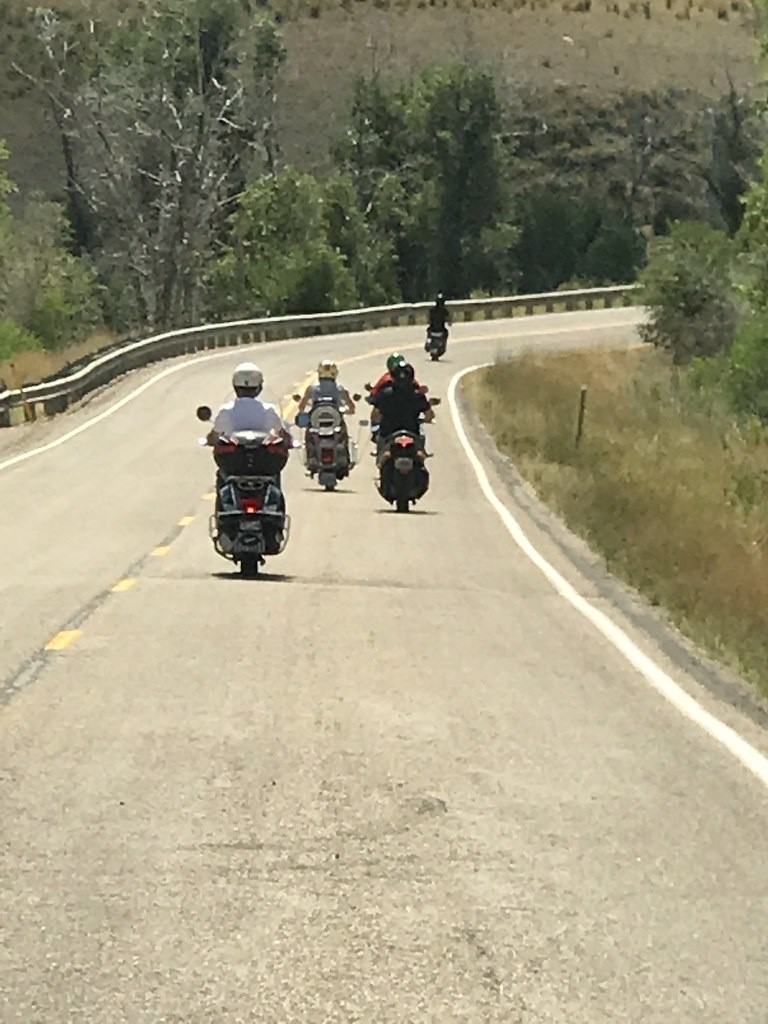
[425,319,456,363]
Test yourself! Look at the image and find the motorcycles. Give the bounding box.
[294,388,361,490]
[195,404,302,578]
[365,395,443,517]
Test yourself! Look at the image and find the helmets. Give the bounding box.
[387,354,405,371]
[393,361,414,384]
[318,360,337,379]
[233,362,263,387]
[435,294,446,304]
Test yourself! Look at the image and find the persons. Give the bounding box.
[427,294,452,341]
[299,360,356,457]
[370,353,433,456]
[206,362,291,513]
